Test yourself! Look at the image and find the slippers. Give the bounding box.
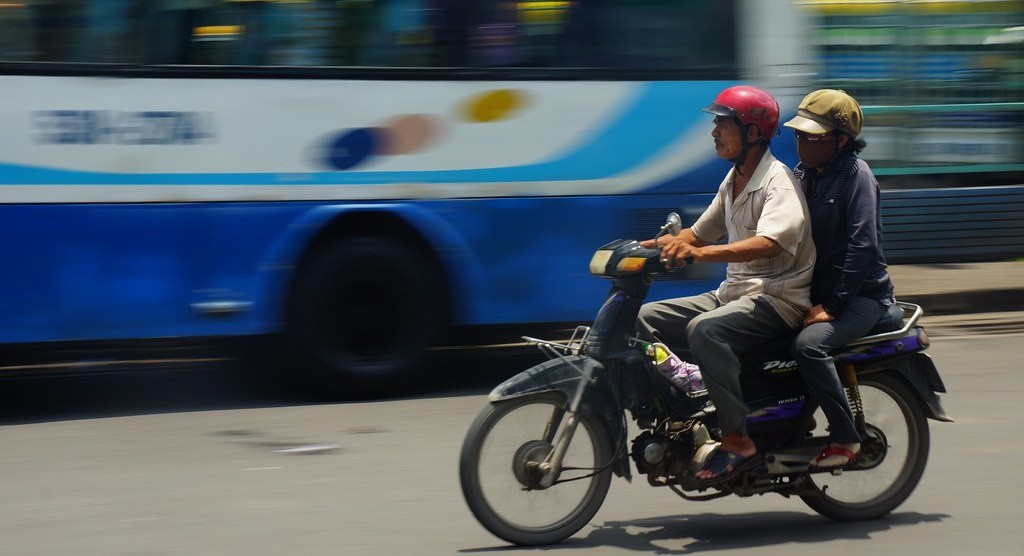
[693,448,764,486]
[808,442,867,472]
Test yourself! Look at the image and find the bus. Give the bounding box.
[0,0,826,403]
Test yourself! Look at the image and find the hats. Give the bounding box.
[782,89,863,139]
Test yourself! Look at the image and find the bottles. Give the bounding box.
[642,342,668,363]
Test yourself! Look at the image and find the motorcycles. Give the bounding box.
[459,207,957,548]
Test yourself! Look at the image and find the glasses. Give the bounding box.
[793,130,834,144]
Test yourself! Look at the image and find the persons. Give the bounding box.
[637,86,896,483]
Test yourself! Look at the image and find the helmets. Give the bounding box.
[701,86,779,141]
